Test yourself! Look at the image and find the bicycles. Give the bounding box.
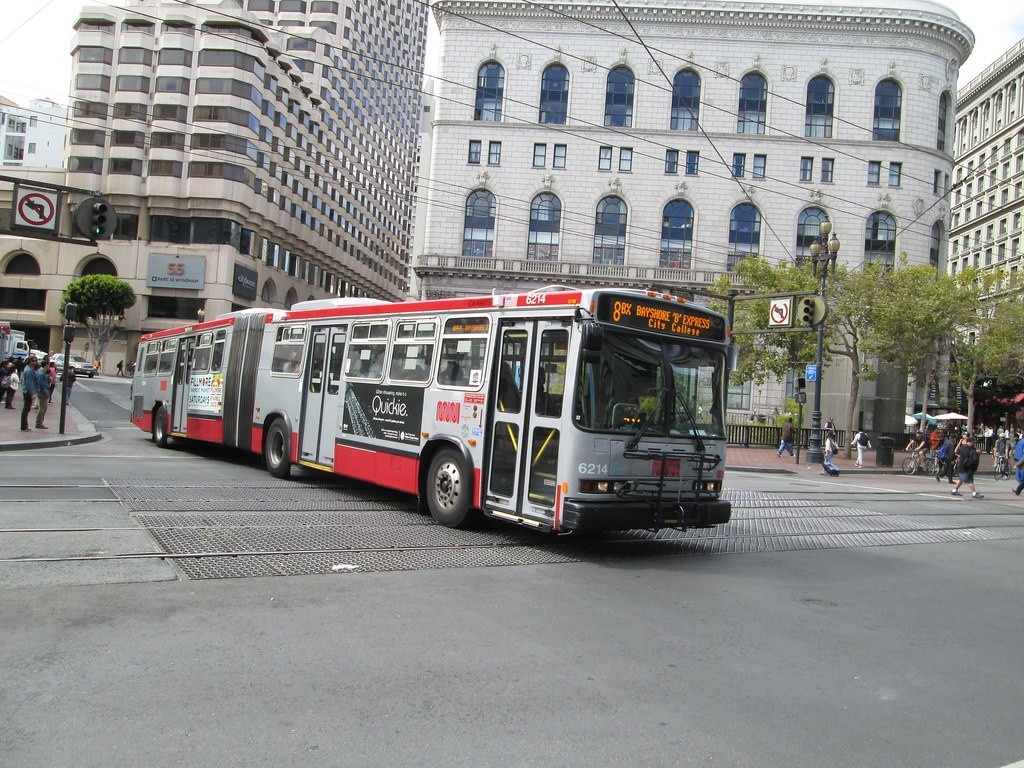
[993,456,1013,481]
[901,449,960,477]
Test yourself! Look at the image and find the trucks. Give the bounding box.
[0,321,50,367]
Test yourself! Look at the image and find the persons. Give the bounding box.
[93,359,100,373]
[696,404,704,422]
[777,417,796,459]
[751,410,757,421]
[59,366,76,405]
[905,430,928,469]
[820,417,838,475]
[851,427,871,468]
[929,422,1024,499]
[116,360,135,378]
[0,355,58,432]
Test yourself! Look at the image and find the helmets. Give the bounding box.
[917,430,923,434]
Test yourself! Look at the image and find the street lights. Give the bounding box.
[804,215,842,463]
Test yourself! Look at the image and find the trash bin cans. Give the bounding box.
[875,436,894,467]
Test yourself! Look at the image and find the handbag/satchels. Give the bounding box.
[829,438,838,455]
[117,364,119,368]
[940,446,949,460]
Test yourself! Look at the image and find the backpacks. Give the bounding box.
[962,450,979,471]
[2,376,11,386]
[858,432,868,446]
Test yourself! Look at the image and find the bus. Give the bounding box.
[131,284,734,543]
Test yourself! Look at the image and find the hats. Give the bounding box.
[999,433,1005,437]
[967,437,979,443]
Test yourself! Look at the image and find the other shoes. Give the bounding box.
[36,426,48,429]
[1012,488,1019,495]
[949,481,956,484]
[5,406,15,410]
[972,492,984,498]
[952,489,962,496]
[935,476,940,483]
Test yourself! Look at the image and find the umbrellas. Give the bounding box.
[905,415,918,425]
[930,412,968,419]
[913,412,932,420]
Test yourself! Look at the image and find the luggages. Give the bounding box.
[822,459,839,476]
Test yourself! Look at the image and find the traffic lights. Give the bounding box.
[92,202,107,236]
[795,378,807,402]
[804,299,815,324]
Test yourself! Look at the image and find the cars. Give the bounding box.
[50,353,96,378]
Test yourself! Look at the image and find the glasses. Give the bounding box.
[32,360,37,362]
